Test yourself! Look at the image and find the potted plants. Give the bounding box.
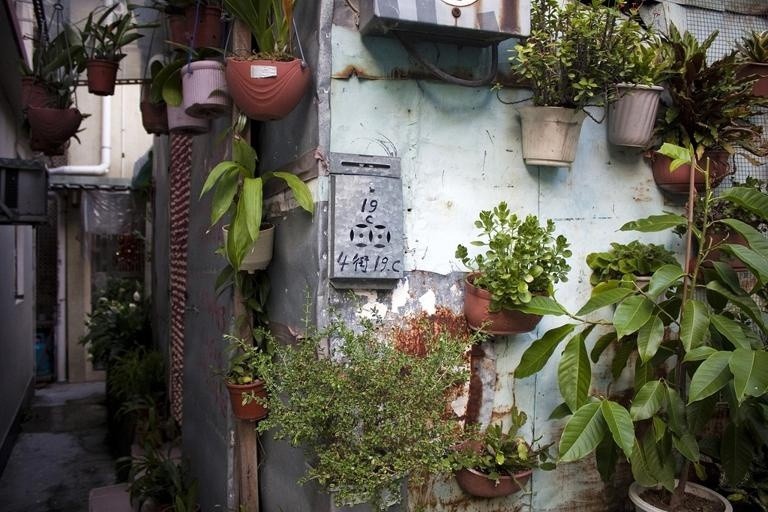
[17,1,315,511]
